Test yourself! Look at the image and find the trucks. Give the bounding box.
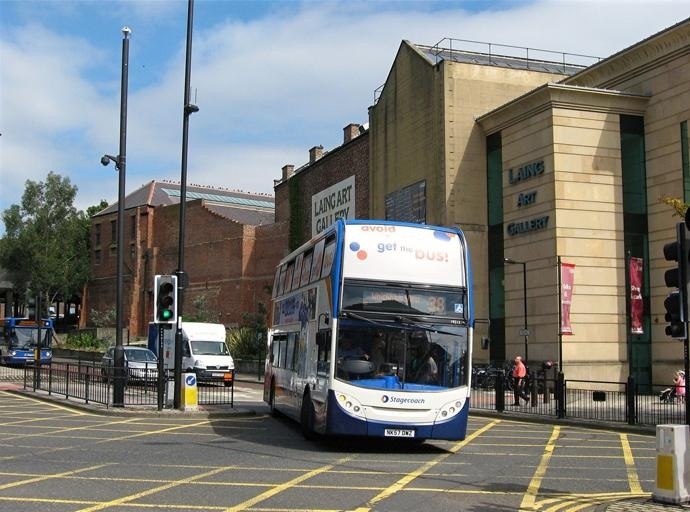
[146,319,236,388]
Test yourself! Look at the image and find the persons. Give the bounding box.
[671,370,685,405]
[511,356,530,406]
[415,348,438,384]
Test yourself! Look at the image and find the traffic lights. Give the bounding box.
[35,296,47,320]
[662,222,689,341]
[154,274,177,323]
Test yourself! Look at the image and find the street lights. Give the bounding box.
[502,258,531,404]
[100,24,135,409]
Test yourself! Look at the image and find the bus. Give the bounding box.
[0,317,51,367]
[262,215,495,445]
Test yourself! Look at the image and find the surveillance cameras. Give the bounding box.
[100,156,110,166]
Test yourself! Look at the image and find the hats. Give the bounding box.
[680,371,685,376]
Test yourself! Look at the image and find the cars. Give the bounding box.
[100,347,160,387]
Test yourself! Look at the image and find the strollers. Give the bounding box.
[658,384,678,404]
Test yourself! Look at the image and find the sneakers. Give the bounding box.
[526,397,530,405]
[510,404,520,406]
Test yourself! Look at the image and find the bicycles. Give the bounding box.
[446,362,544,395]
